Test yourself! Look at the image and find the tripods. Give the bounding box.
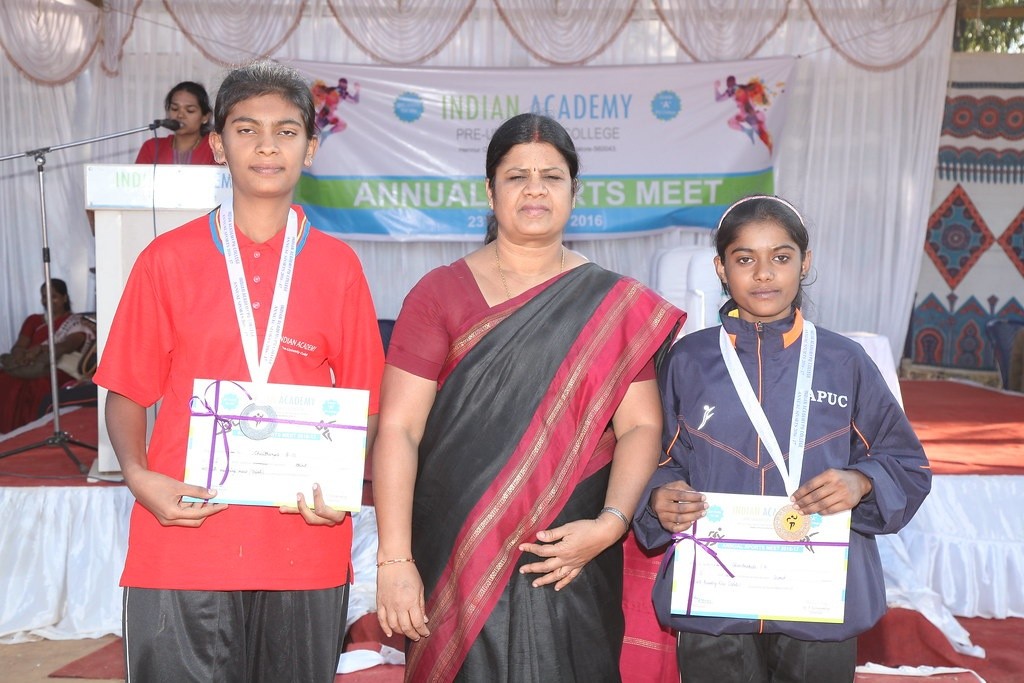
[0,122,163,474]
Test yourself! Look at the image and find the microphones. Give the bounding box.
[155,119,179,131]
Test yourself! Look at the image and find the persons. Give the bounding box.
[635,195,932,683]
[372,117,688,683]
[89,60,385,683]
[136,81,223,165]
[1,279,94,434]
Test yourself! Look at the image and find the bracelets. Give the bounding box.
[376,558,415,567]
[600,507,629,531]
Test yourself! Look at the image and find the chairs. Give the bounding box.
[986,320,1024,393]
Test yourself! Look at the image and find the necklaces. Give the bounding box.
[493,240,565,298]
